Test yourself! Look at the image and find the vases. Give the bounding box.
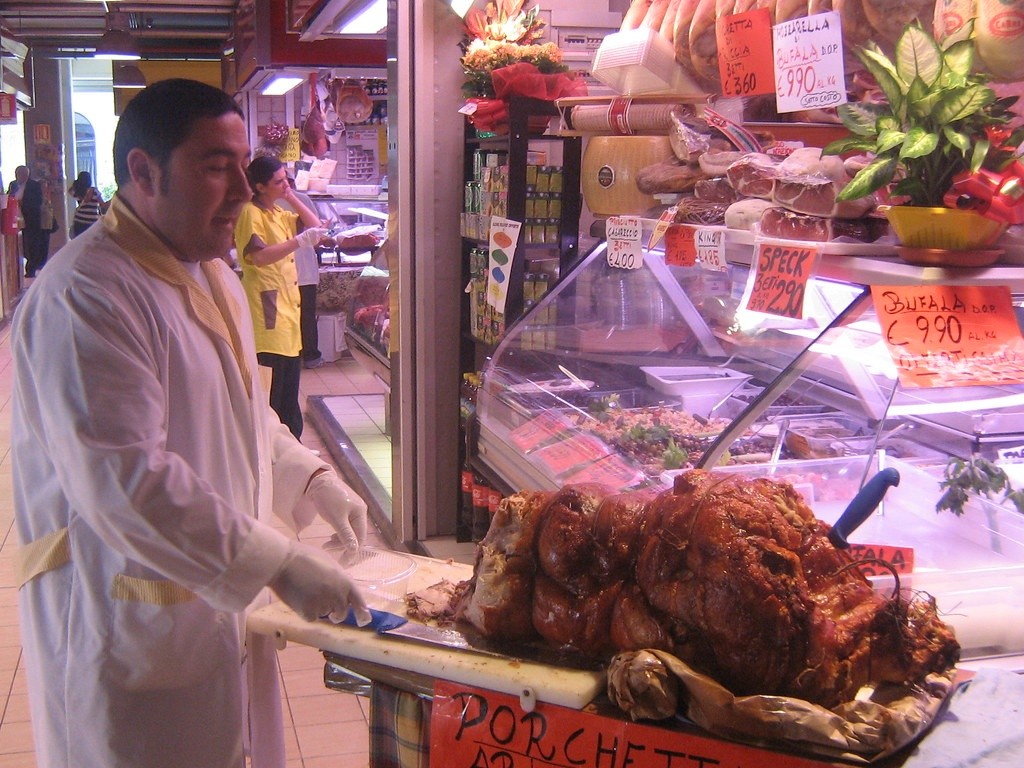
[465,93,552,136]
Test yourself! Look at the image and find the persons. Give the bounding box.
[8,78,370,768]
[274,171,325,368]
[35,178,52,270]
[235,157,340,443]
[68,171,112,240]
[7,163,43,277]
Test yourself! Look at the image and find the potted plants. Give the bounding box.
[820,14,1023,268]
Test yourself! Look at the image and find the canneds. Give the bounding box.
[464,147,509,280]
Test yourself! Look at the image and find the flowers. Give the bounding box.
[453,0,565,100]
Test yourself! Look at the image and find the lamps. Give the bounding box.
[93,11,141,61]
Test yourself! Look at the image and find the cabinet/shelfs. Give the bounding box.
[459,98,582,406]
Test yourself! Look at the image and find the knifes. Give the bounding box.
[321,603,610,670]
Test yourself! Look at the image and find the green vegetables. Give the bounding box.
[588,392,688,471]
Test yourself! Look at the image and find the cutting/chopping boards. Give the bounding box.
[621,214,900,257]
[250,547,609,708]
